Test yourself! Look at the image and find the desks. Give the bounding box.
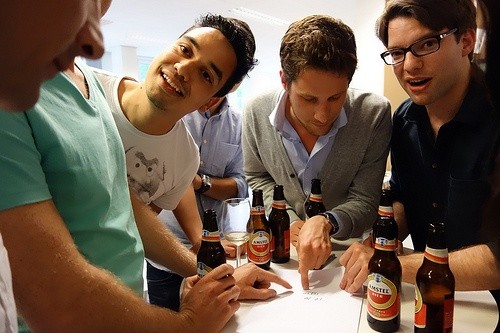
[227,237,500,333]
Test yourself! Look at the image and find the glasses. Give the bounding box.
[380,28,458,65]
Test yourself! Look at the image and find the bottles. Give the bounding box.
[305,178,326,221]
[196,208,226,282]
[365,189,403,333]
[268,185,290,264]
[413,222,455,333]
[245,189,271,272]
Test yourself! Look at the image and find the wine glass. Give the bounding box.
[221,198,254,268]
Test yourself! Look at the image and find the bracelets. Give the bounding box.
[196,174,211,194]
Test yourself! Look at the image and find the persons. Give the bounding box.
[0,61,293,333]
[339,0,500,292]
[92,11,258,256]
[145,96,249,313]
[0,0,111,333]
[241,15,391,290]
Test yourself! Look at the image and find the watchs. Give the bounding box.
[320,212,339,236]
